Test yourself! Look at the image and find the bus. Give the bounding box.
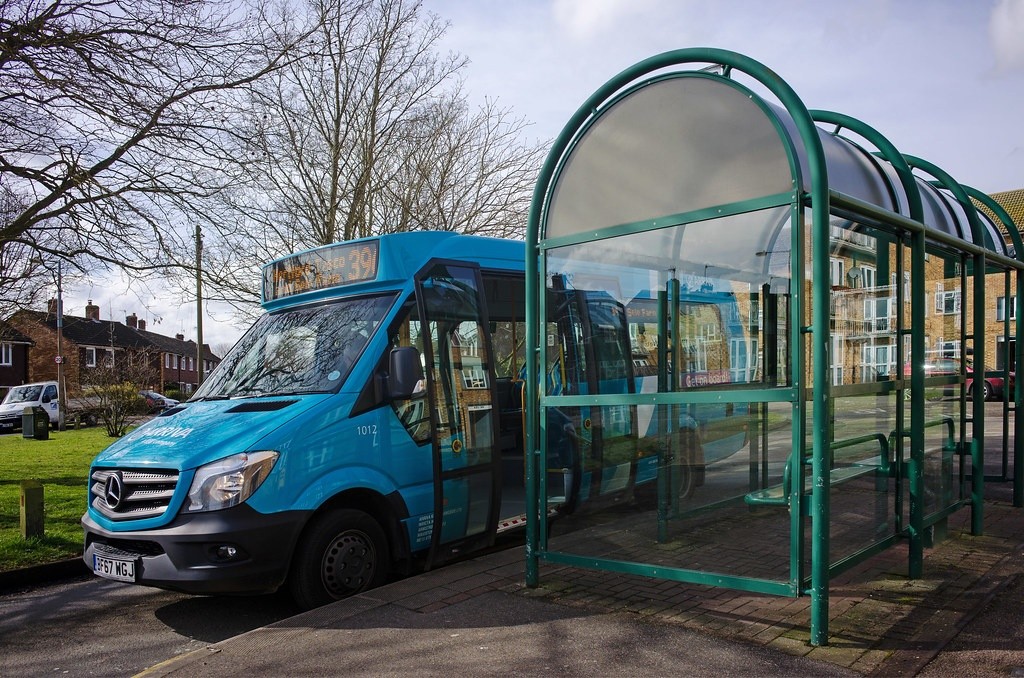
[79,230,749,608]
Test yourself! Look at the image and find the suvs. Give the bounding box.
[888,360,1018,402]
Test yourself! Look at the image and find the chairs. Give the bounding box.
[388,347,425,399]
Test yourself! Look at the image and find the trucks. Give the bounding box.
[0,377,112,433]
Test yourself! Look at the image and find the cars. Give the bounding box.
[120,390,177,414]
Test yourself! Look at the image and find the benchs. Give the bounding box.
[851,418,955,479]
[744,433,894,505]
